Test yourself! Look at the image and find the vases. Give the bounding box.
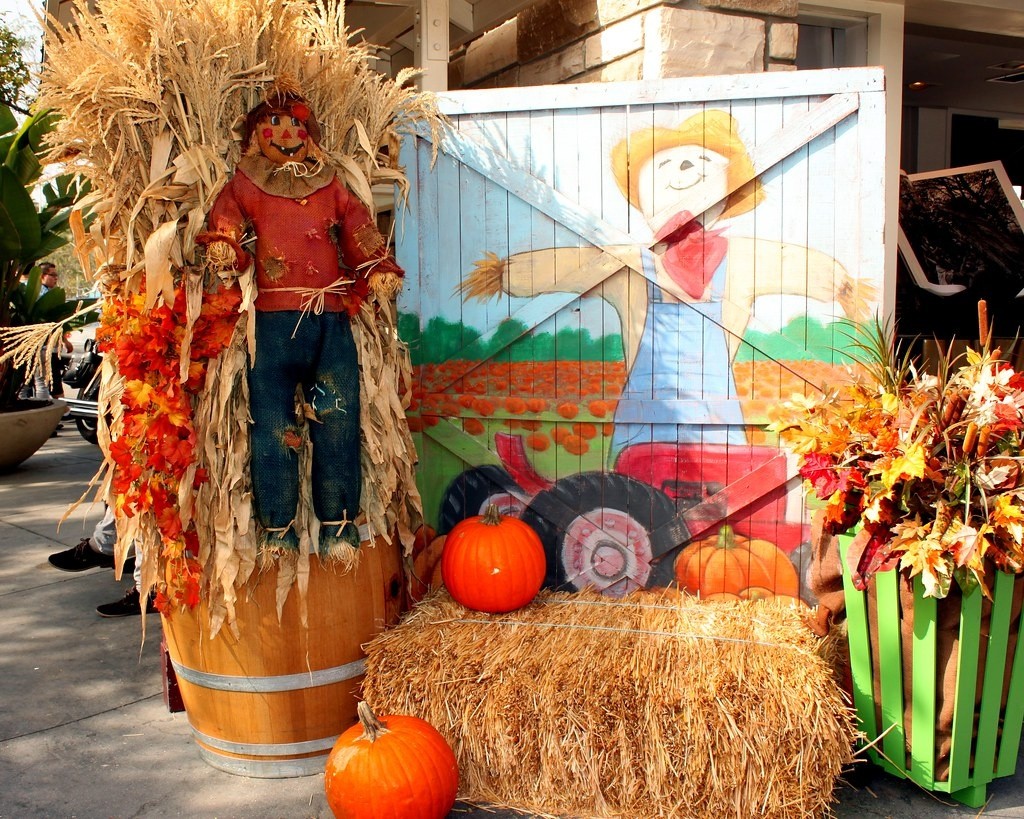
[831,535,1023,809]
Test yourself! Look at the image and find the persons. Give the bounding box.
[37,263,73,421]
[47,480,160,618]
[191,93,406,572]
[15,259,63,438]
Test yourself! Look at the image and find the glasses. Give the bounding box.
[46,272,62,278]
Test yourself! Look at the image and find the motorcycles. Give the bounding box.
[57,338,103,445]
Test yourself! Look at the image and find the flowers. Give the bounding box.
[768,300,1022,600]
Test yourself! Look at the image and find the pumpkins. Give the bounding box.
[674,526,799,606]
[440,504,547,613]
[325,700,460,819]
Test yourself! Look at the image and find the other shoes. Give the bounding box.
[98,587,148,615]
[46,541,111,570]
[48,409,76,439]
